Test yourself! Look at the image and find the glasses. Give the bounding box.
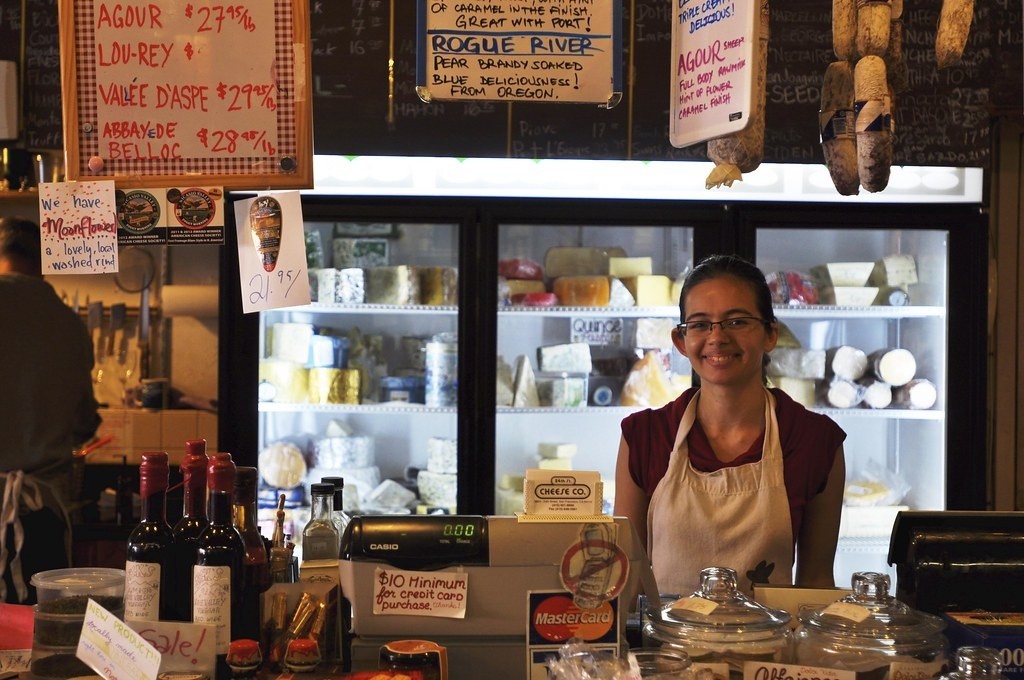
[676,316,771,337]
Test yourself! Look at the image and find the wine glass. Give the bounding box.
[108,350,138,411]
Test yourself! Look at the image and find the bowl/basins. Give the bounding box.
[808,261,875,286]
[821,286,880,307]
[31,567,123,680]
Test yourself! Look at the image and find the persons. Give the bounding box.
[0,217,100,604]
[612,255,848,614]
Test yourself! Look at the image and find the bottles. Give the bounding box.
[421,331,457,407]
[627,566,1007,680]
[379,376,421,405]
[141,377,171,409]
[124,440,350,677]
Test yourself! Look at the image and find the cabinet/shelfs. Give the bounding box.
[225,192,989,598]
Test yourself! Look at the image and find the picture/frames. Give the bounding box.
[57,1,314,192]
